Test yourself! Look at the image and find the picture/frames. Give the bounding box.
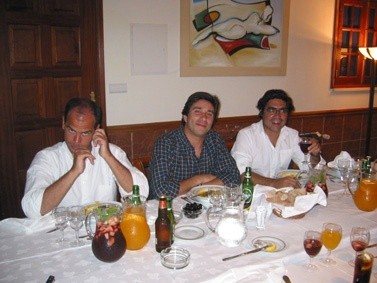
[180,0,290,77]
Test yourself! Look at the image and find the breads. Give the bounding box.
[266,188,306,206]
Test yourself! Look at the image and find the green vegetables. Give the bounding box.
[97,207,117,221]
[311,169,324,183]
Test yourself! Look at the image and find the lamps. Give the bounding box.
[358,47,377,157]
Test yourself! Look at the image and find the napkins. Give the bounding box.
[327,150,357,169]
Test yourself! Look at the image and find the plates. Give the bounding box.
[250,235,286,253]
[173,224,204,240]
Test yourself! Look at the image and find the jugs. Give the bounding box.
[120,196,151,250]
[346,171,377,212]
[85,203,126,263]
[206,198,248,246]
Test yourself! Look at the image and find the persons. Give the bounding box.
[149,92,240,200]
[21,99,149,217]
[231,90,326,189]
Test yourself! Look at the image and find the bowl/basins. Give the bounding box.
[186,185,228,205]
[181,202,204,218]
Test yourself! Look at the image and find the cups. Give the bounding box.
[224,183,240,202]
[352,252,373,283]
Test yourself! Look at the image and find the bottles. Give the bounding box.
[132,185,141,205]
[242,167,253,205]
[358,156,372,183]
[154,194,173,254]
[164,196,175,244]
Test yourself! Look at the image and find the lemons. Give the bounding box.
[266,241,277,252]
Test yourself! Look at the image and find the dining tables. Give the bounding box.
[0,161,377,283]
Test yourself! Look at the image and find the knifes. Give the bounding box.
[222,244,269,262]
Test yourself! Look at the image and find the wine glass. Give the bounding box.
[298,136,312,165]
[52,206,85,247]
[303,223,370,273]
[336,155,363,190]
